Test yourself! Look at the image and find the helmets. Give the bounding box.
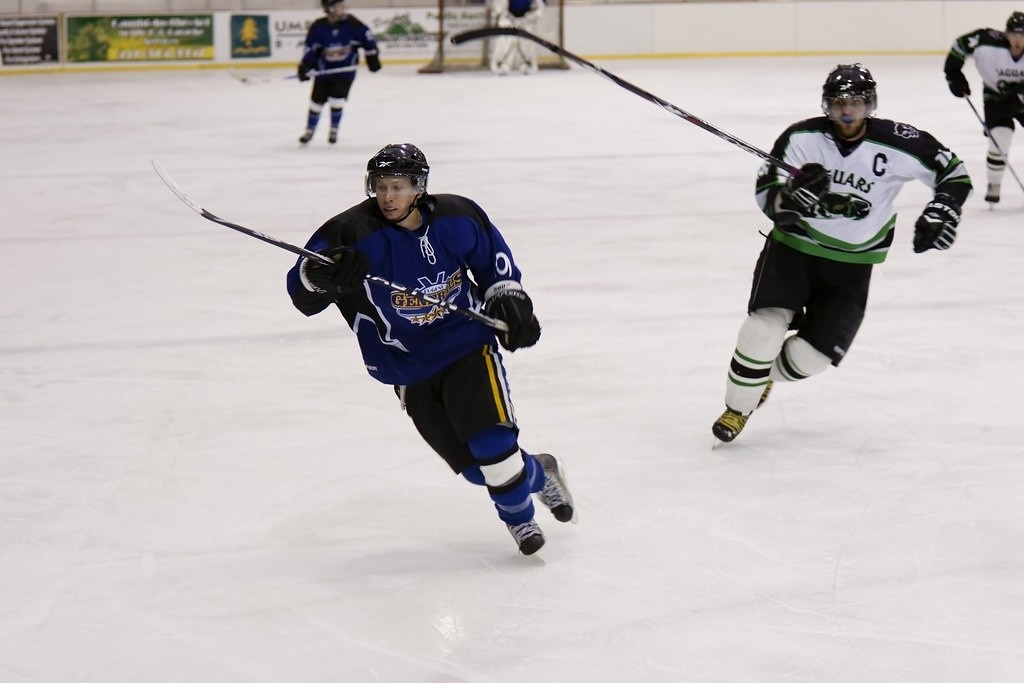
[366,142,429,186]
[1007,12,1024,32]
[822,63,875,104]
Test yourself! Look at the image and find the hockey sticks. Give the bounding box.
[963,95,1024,190]
[227,63,367,86]
[151,158,508,331]
[451,26,800,177]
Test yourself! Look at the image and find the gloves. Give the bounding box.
[309,245,368,307]
[299,63,309,82]
[366,52,381,72]
[914,194,962,254]
[484,295,541,353]
[946,72,970,97]
[784,162,829,210]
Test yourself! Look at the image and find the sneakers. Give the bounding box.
[985,182,1000,210]
[755,380,773,409]
[330,127,337,144]
[712,405,750,454]
[532,453,572,523]
[301,132,311,145]
[507,518,545,566]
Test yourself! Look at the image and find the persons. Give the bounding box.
[299,0,382,144]
[712,63,973,451]
[945,10,1024,212]
[490,0,545,75]
[285,143,578,566]
